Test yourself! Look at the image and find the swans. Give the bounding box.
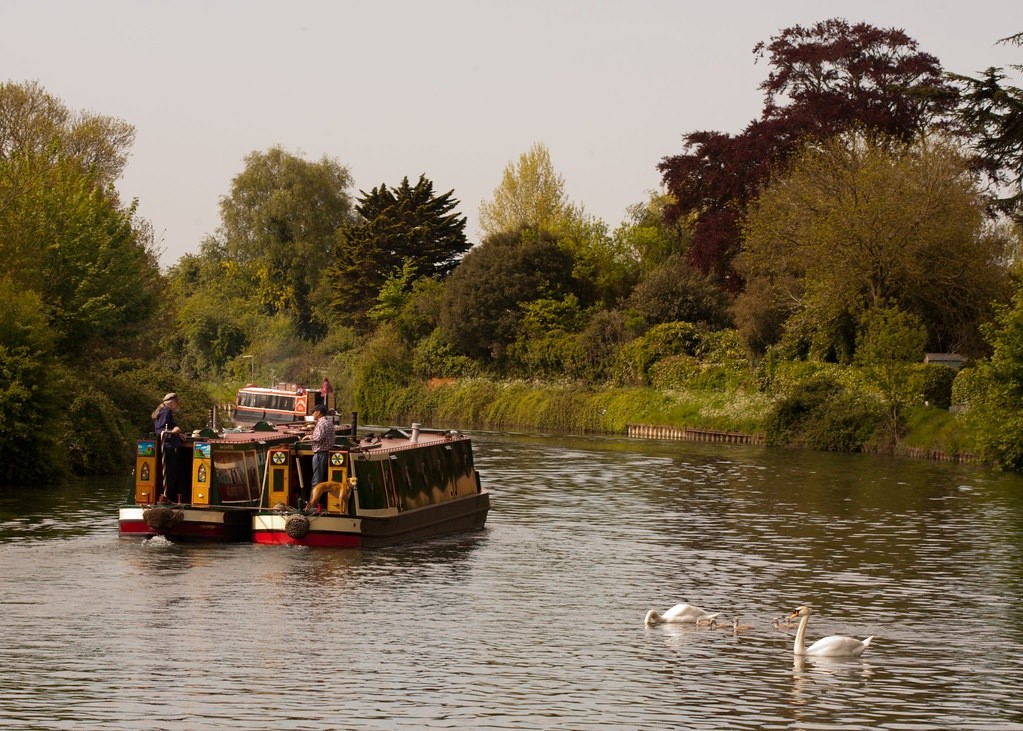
[643,603,756,632]
[771,605,874,657]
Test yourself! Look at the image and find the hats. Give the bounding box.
[313,404,327,411]
[163,393,177,401]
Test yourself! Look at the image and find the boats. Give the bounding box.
[114,412,347,543]
[228,377,338,427]
[248,410,492,548]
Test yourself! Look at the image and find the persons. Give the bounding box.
[301,403,336,508]
[151,392,187,503]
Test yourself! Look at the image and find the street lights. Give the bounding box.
[242,353,256,384]
